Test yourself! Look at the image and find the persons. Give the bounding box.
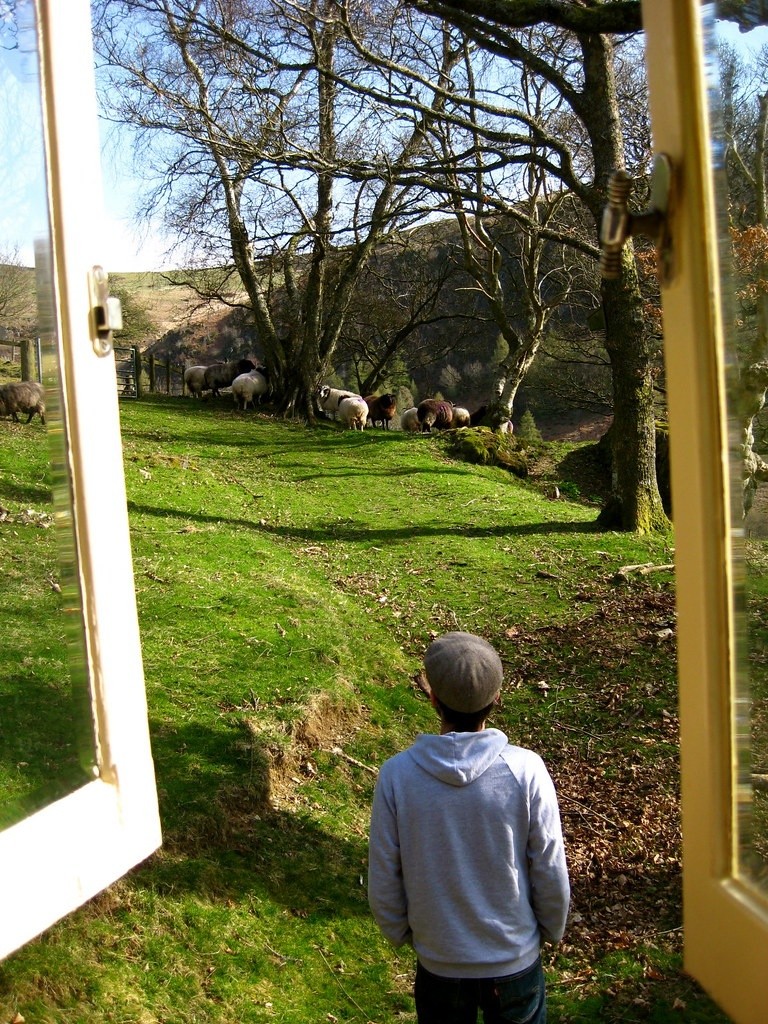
[368,632,572,1024]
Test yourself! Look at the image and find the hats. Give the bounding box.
[425,632,502,713]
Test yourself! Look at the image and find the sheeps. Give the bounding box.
[183,359,272,411]
[0,380,47,425]
[318,382,514,436]
